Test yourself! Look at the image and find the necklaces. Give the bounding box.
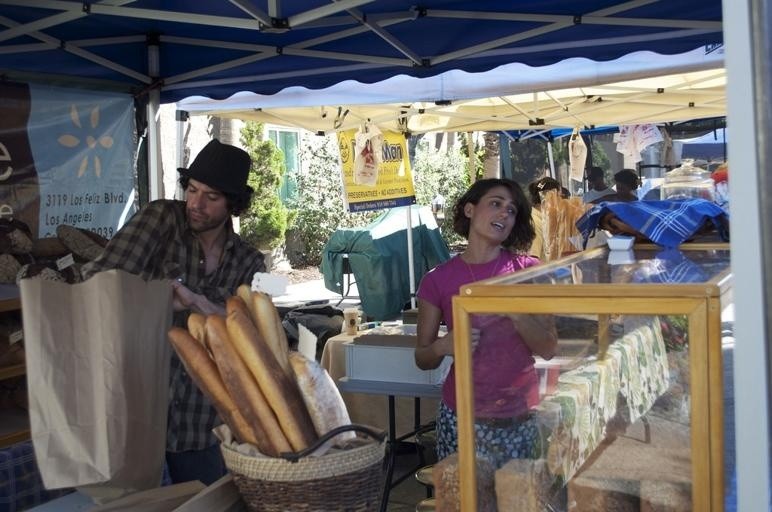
[463,254,502,282]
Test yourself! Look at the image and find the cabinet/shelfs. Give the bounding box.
[0,281,31,450]
[452,242,734,512]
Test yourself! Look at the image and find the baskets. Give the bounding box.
[601,211,724,243]
[219,422,392,512]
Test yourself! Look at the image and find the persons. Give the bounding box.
[517,177,562,258]
[582,168,616,203]
[590,169,640,204]
[561,188,571,199]
[415,178,557,498]
[81,139,265,486]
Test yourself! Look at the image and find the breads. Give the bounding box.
[167,283,356,456]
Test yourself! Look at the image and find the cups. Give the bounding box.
[343,308,358,335]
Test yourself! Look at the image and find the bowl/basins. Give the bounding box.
[606,235,636,251]
[606,249,637,264]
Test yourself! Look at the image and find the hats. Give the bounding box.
[177,138,252,197]
[584,167,603,181]
[535,176,571,197]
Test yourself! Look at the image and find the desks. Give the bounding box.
[659,178,716,202]
[336,253,361,308]
[341,377,444,512]
